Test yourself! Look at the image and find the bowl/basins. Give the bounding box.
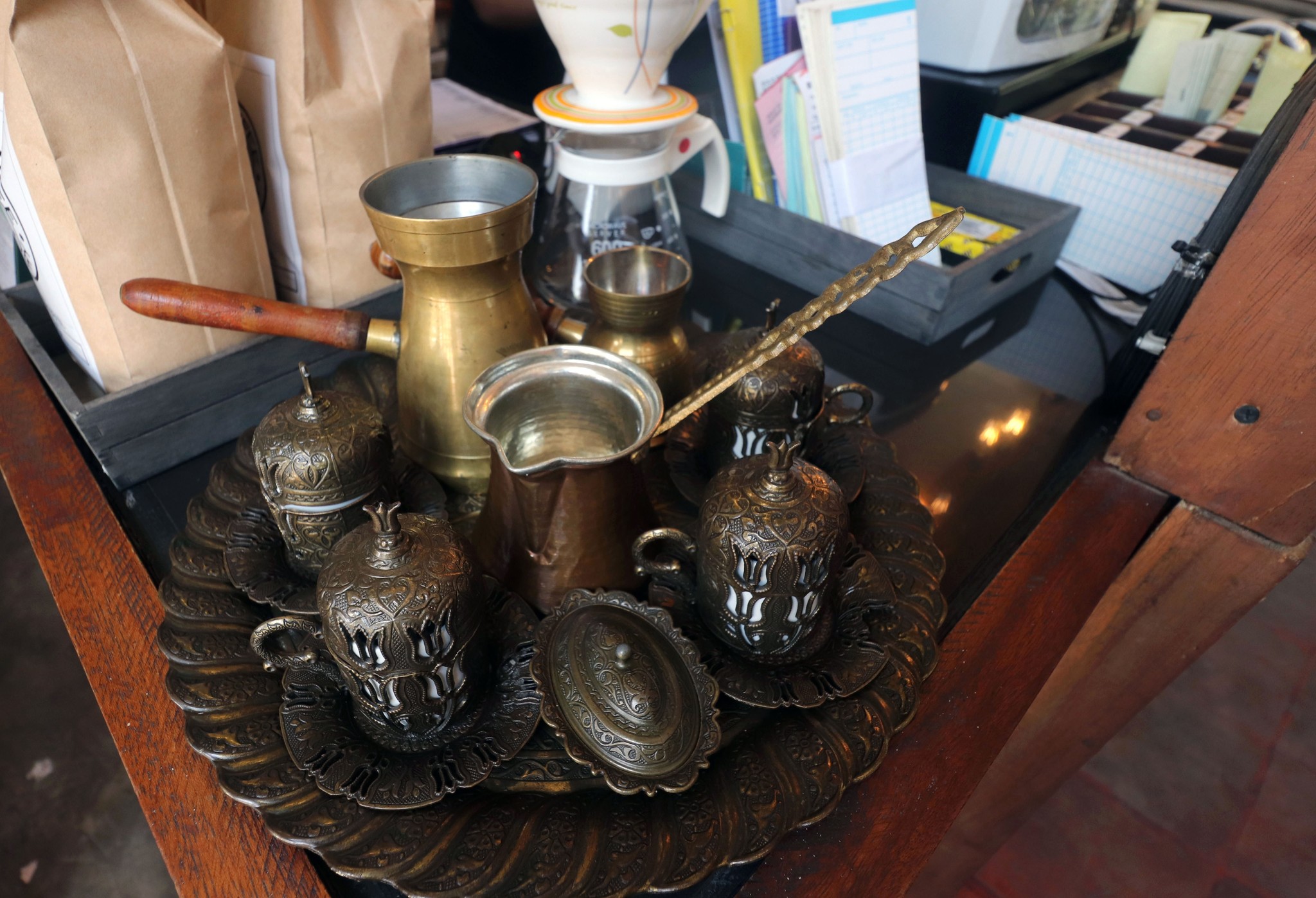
[532,0,713,94]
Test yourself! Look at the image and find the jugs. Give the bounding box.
[531,88,733,315]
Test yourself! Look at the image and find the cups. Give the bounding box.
[120,152,548,496]
[533,247,700,410]
[461,202,968,618]
[253,361,392,580]
[636,440,847,663]
[250,501,476,740]
[695,299,875,476]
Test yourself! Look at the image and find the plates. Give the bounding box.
[154,333,948,897]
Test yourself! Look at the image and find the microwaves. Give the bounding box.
[916,0,1119,73]
